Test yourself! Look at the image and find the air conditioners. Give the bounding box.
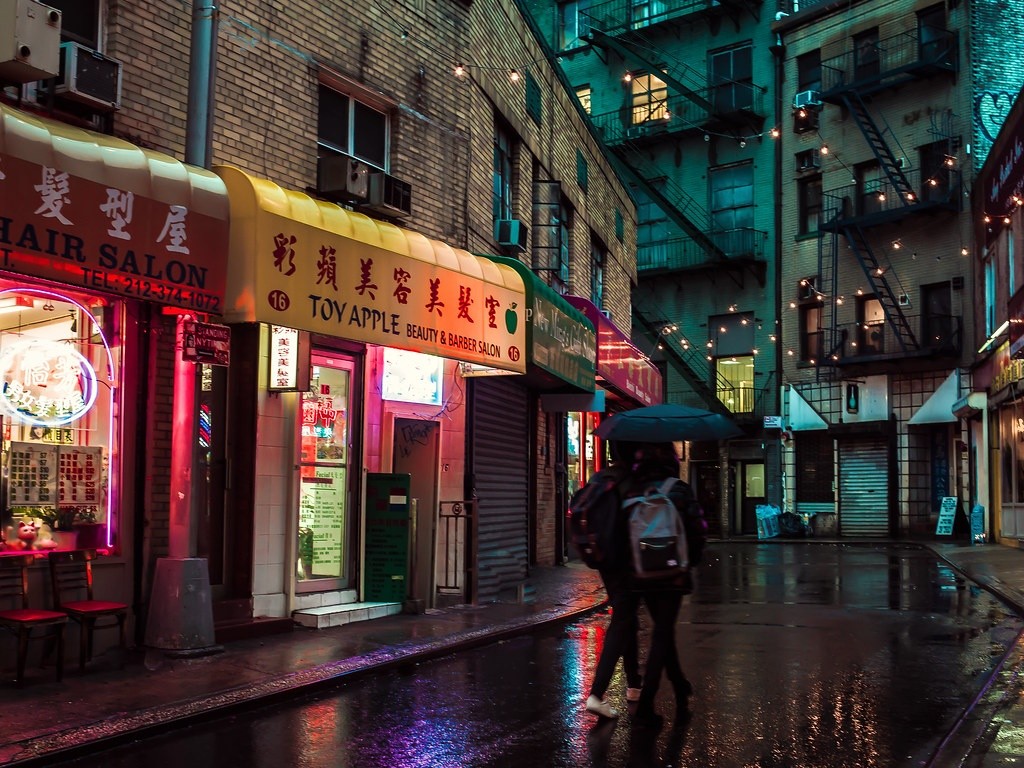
[360,171,411,220]
[38,40,122,116]
[493,219,529,254]
[794,90,823,108]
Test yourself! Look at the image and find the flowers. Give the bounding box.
[73,510,98,523]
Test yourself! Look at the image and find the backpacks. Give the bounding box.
[629,478,689,578]
[570,469,629,567]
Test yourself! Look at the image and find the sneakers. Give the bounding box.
[626,687,642,701]
[586,695,618,719]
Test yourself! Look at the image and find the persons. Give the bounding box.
[29,418,53,443]
[623,441,705,728]
[584,439,647,720]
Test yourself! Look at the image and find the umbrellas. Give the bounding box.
[589,405,744,441]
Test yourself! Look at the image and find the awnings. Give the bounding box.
[473,256,595,396]
[0,102,231,317]
[536,294,663,410]
[207,166,526,379]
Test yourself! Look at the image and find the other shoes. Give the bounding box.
[635,703,662,721]
[676,681,691,708]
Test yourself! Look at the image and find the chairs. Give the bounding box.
[0,553,70,690]
[47,550,131,662]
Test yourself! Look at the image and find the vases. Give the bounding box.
[67,522,102,549]
